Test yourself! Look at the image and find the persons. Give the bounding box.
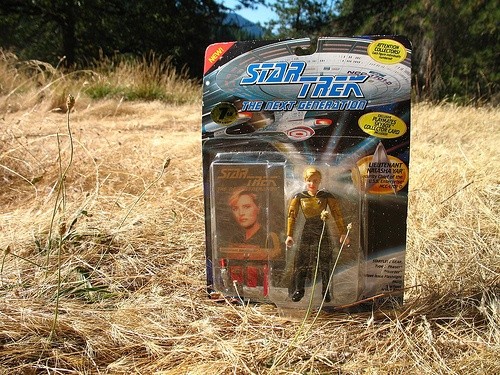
[228,188,281,251]
[284,166,350,302]
